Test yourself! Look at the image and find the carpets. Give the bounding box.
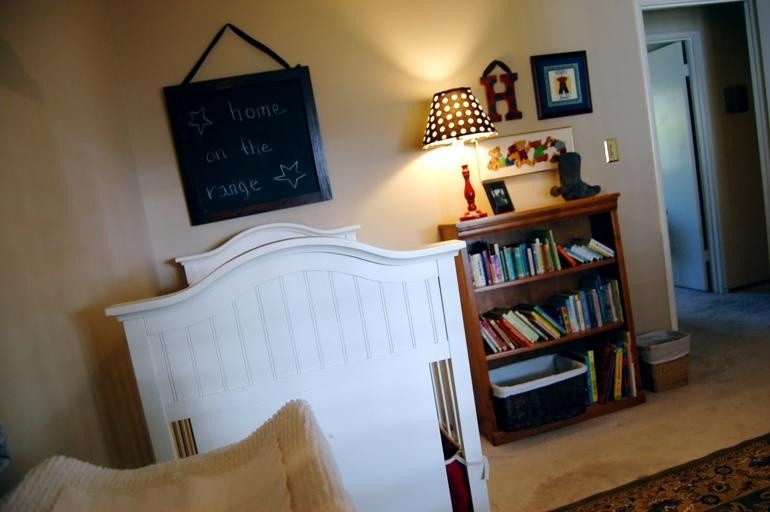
[550,431,768,511]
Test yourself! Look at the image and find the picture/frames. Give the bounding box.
[482,179,514,215]
[529,50,594,120]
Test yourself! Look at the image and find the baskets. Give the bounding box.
[487,353,588,432]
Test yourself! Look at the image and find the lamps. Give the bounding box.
[416,82,499,219]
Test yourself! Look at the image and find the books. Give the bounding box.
[467,229,639,408]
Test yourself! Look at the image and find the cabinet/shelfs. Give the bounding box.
[437,192,650,448]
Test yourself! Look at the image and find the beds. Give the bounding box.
[102,214,498,512]
